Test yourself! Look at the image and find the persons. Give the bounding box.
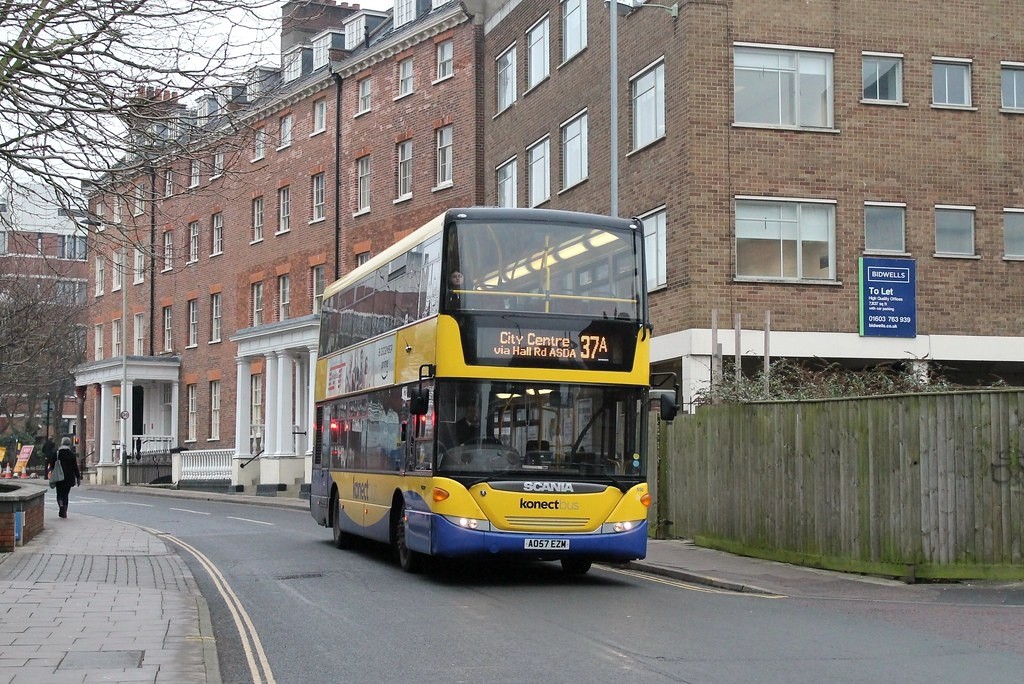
[50,437,80,518]
[42,436,57,479]
[456,416,481,447]
[448,268,474,310]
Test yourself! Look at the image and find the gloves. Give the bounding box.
[77,478,81,487]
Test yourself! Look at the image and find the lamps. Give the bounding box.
[604,0,678,20]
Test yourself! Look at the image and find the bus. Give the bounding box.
[310,207,681,576]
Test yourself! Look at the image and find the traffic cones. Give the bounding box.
[20,463,31,479]
[4,462,12,479]
[48,463,52,480]
[0,461,5,479]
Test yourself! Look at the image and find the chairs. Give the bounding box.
[527,439,550,453]
[474,281,622,314]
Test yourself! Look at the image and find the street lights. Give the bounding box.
[78,217,127,487]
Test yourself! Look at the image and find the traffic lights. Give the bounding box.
[74,436,80,447]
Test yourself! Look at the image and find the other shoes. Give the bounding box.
[59,507,67,519]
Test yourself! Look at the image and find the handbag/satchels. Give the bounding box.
[49,451,65,489]
